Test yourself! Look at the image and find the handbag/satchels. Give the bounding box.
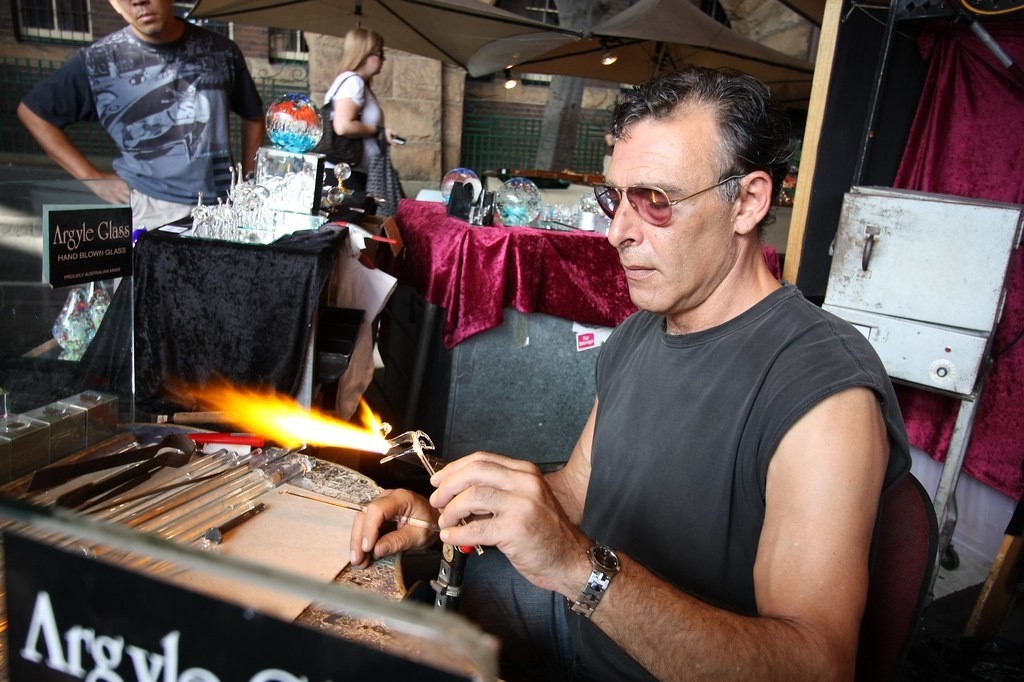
[309,73,366,169]
[366,145,406,217]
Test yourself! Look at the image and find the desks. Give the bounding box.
[115,423,408,653]
[132,187,379,420]
[395,199,778,472]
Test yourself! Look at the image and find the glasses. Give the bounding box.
[370,50,383,58]
[594,175,746,226]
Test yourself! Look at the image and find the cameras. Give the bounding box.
[393,136,405,145]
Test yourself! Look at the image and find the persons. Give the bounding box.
[347,61,910,682]
[20,0,268,236]
[321,29,411,232]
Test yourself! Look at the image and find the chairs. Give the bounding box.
[416,190,444,203]
[855,472,939,682]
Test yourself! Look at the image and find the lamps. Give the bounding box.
[504,68,517,89]
[599,37,618,66]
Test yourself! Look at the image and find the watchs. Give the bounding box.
[572,537,622,622]
